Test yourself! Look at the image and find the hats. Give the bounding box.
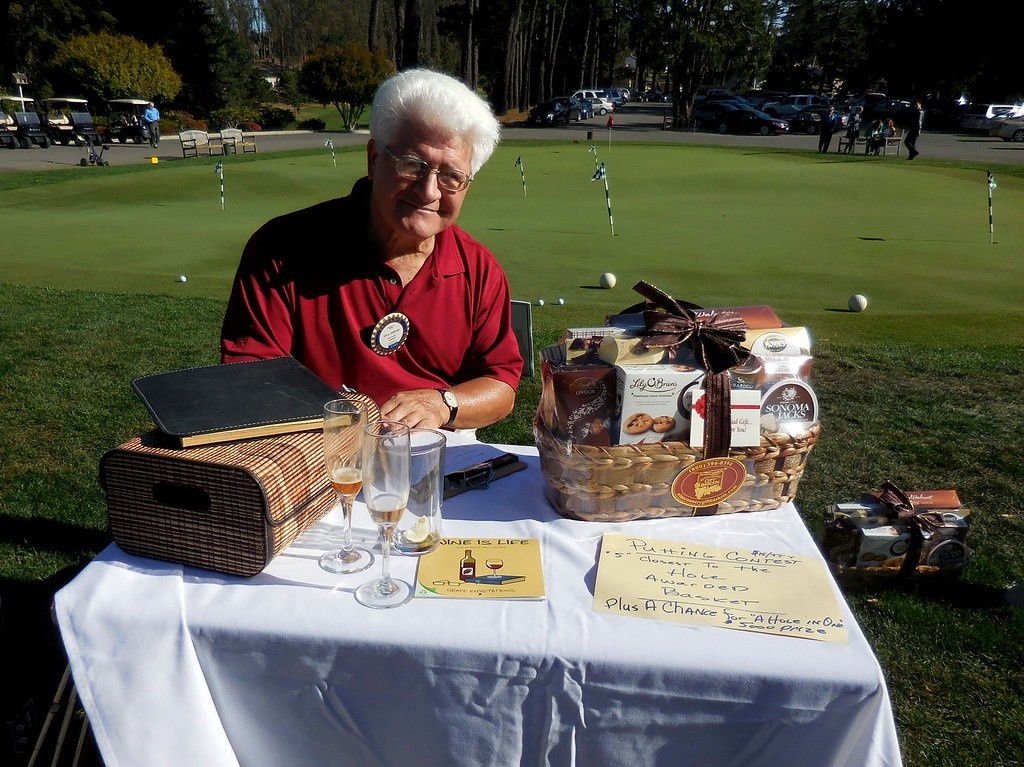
[828,106,834,110]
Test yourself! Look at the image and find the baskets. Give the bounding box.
[533,411,821,523]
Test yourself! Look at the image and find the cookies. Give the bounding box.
[858,552,889,561]
[590,417,602,434]
[623,413,677,435]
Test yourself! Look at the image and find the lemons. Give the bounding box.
[402,514,430,543]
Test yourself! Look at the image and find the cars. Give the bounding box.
[526,88,673,126]
[691,82,1024,142]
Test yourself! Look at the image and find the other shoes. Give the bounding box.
[907,151,919,161]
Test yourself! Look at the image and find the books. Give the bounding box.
[416,538,546,601]
[132,356,361,448]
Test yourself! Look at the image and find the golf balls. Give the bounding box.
[599,272,616,289]
[180,275,187,282]
[848,295,868,311]
[557,298,564,305]
[538,300,545,306]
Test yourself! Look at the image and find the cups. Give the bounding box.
[390,428,447,556]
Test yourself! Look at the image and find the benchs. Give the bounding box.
[47,114,73,130]
[220,128,257,156]
[0,113,18,131]
[838,129,905,156]
[178,130,225,159]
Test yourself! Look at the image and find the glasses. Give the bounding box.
[385,147,473,192]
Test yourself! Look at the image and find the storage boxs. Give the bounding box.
[98,393,384,577]
[816,490,969,569]
[540,305,814,446]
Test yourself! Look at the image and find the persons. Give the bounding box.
[218,69,523,441]
[844,106,863,153]
[864,118,896,155]
[144,103,160,146]
[819,107,836,154]
[904,101,924,159]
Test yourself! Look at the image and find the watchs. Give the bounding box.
[435,388,459,429]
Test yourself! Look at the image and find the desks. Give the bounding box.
[25,444,906,767]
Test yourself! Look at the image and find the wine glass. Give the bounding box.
[355,422,414,609]
[318,399,375,574]
